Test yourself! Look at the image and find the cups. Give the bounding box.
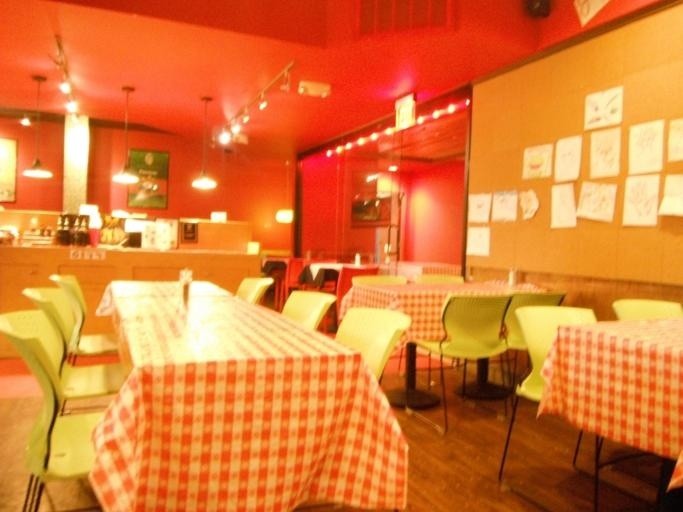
[87,228,99,247]
[126,231,142,247]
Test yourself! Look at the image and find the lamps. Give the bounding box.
[110,87,137,184]
[21,74,51,179]
[217,68,291,146]
[192,96,218,189]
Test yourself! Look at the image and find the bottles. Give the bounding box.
[506,266,516,288]
[178,266,193,313]
[353,252,361,268]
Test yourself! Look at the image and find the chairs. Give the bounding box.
[503,288,566,405]
[611,297,681,321]
[1,309,105,511]
[280,290,337,328]
[408,291,513,434]
[22,287,125,416]
[334,306,412,378]
[255,255,465,324]
[47,273,118,366]
[496,305,648,509]
[235,275,274,304]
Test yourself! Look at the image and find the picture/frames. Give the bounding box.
[0,137,18,204]
[127,147,170,208]
